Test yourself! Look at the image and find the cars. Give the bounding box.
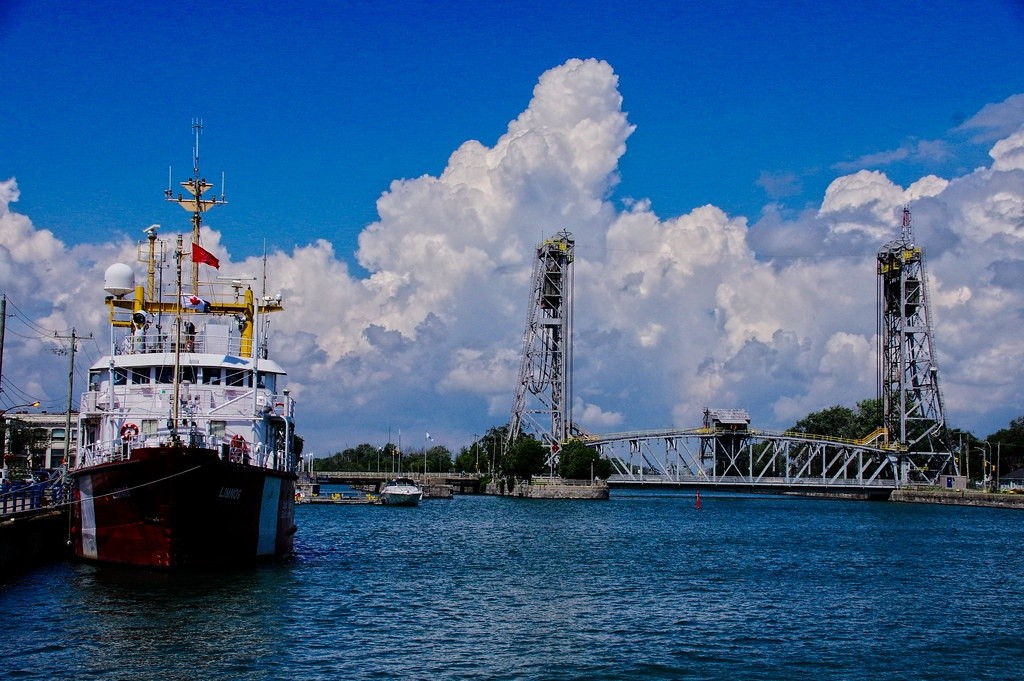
[14,473,34,485]
[0,469,9,485]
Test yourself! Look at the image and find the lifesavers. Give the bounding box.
[231,435,246,454]
[272,402,285,416]
[120,424,138,442]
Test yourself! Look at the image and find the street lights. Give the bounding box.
[985,441,991,478]
[974,447,985,475]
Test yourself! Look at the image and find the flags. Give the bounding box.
[192,243,220,270]
[379,446,383,451]
[181,293,206,311]
[426,432,433,441]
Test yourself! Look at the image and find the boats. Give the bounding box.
[420,484,453,498]
[380,477,423,505]
[63,114,302,575]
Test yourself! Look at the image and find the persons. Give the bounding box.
[462,470,465,478]
